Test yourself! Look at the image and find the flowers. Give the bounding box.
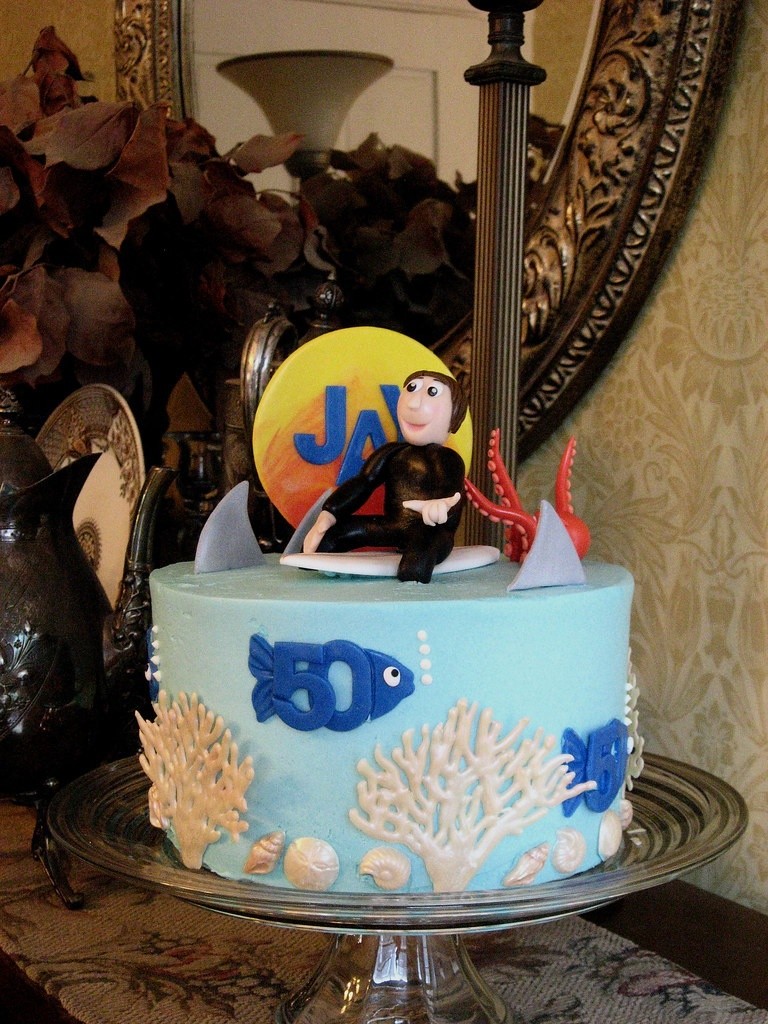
[1,22,340,491]
[303,112,564,344]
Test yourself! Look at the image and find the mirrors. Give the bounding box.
[112,1,745,531]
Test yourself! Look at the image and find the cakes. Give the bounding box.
[133,324,646,894]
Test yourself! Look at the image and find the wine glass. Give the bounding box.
[160,427,224,562]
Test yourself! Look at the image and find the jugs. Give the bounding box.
[0,420,179,797]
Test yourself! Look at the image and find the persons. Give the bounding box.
[301,370,467,583]
